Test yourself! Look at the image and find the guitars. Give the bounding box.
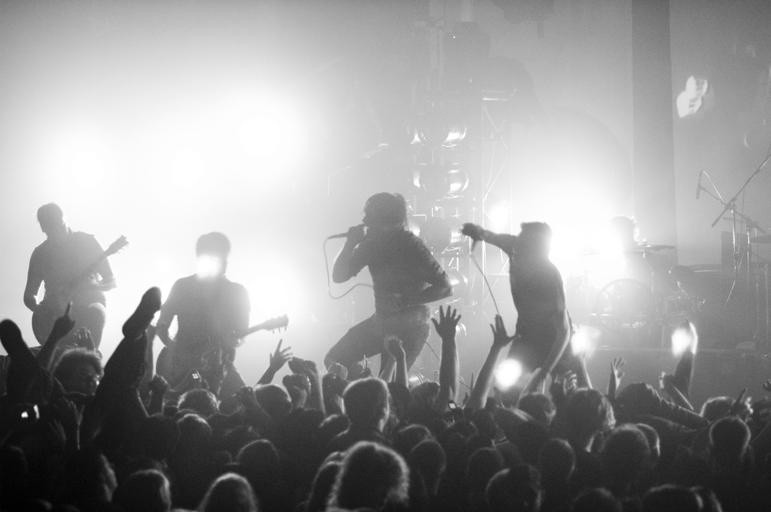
[31,235,127,345]
[156,315,286,389]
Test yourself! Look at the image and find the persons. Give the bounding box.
[608,214,643,273]
[23,202,119,348]
[461,221,592,396]
[156,233,251,397]
[1,286,770,512]
[325,192,455,381]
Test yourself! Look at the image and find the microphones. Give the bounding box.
[332,226,368,238]
[695,170,702,200]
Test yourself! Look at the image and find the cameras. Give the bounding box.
[15,403,39,424]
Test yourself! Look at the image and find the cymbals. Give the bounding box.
[619,244,674,254]
[749,234,769,242]
